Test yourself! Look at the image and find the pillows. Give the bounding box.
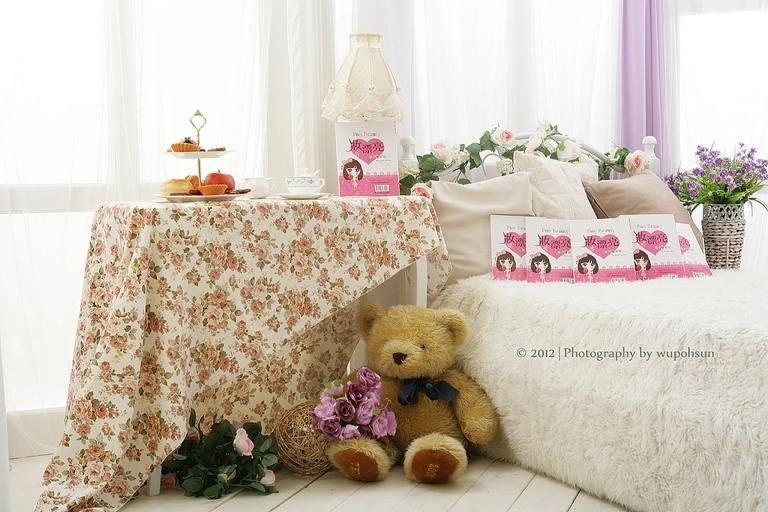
[428,150,703,286]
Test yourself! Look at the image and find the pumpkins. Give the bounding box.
[204,169,235,194]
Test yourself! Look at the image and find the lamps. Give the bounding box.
[316,32,405,124]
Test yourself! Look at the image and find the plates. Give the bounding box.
[280,192,327,199]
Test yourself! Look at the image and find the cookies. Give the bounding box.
[226,189,251,194]
[189,190,202,195]
[208,146,225,151]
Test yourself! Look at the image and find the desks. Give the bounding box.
[34,198,452,512]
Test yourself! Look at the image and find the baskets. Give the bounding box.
[702,220,745,269]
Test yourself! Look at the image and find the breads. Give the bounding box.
[159,175,199,195]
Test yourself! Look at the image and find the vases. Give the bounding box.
[702,202,746,272]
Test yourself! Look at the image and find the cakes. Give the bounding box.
[171,137,201,152]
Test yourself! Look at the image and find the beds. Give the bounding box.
[399,130,768,511]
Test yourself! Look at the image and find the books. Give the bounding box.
[336,119,401,196]
[488,214,713,286]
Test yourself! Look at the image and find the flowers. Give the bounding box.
[664,141,768,217]
[165,358,399,499]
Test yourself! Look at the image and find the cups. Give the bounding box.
[287,175,325,193]
[245,177,278,199]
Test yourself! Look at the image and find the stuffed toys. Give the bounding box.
[324,296,498,485]
